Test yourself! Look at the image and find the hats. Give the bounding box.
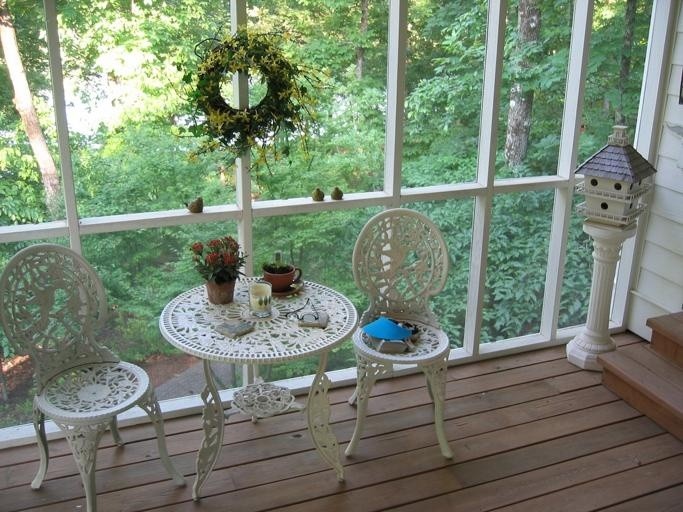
[364,316,421,341]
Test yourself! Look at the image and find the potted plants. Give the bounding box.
[264,261,298,290]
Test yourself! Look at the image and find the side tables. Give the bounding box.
[156,277,356,500]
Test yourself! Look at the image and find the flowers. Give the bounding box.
[191,236,244,280]
[162,21,330,167]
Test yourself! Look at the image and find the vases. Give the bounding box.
[203,276,235,305]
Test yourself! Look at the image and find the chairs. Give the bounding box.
[1,243,187,509]
[340,207,455,472]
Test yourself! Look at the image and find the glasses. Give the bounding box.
[285,297,320,323]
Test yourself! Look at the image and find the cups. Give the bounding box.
[248,279,272,318]
